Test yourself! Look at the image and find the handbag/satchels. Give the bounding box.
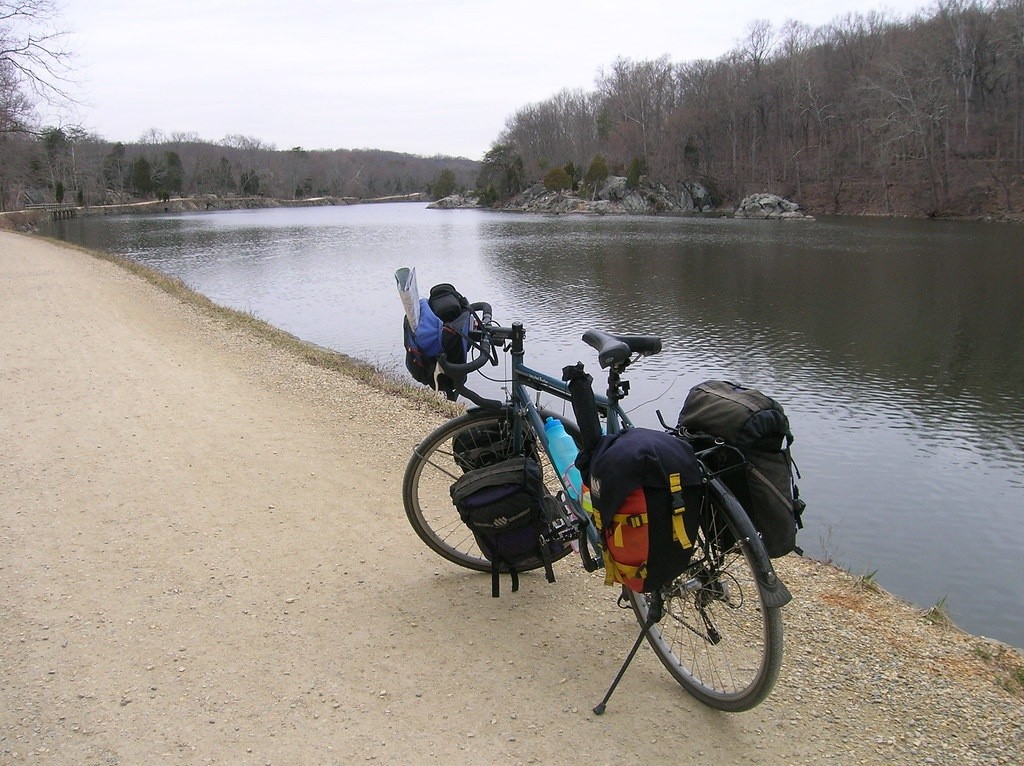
[585,428,705,595]
[450,457,562,597]
[674,379,805,558]
[453,425,540,474]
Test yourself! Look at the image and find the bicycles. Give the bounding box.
[402,302,793,715]
